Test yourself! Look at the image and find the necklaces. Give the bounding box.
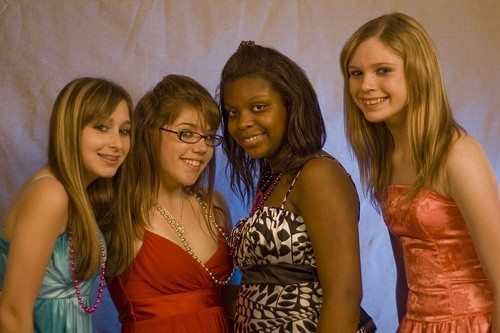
[248,166,283,216]
[68,227,106,314]
[151,186,235,285]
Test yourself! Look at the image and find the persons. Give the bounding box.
[0,77,135,333]
[339,12,500,333]
[214,41,378,333]
[103,74,234,333]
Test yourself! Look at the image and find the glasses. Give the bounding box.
[158,125,223,148]
